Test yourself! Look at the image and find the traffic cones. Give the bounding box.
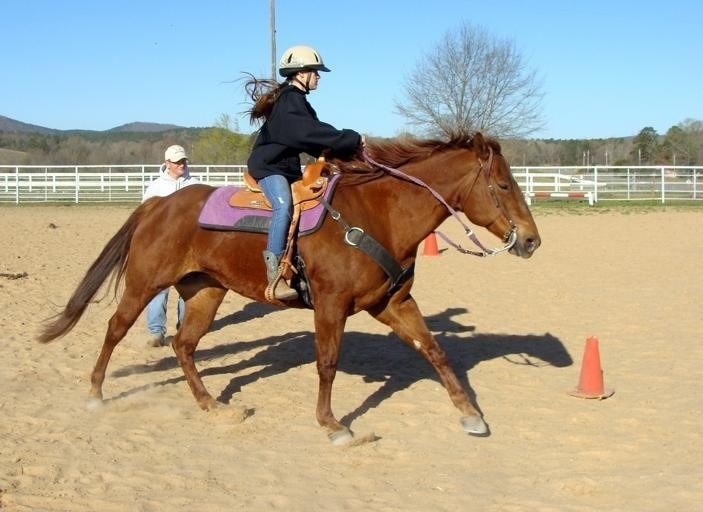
[568,335,615,401]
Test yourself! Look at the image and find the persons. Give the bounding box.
[138,143,199,348]
[240,46,366,303]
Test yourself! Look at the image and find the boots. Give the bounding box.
[258,249,304,304]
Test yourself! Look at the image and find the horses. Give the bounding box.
[35,129,541,447]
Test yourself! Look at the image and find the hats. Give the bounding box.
[164,144,189,164]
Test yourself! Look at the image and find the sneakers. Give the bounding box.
[146,328,168,348]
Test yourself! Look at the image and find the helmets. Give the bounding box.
[278,45,331,77]
